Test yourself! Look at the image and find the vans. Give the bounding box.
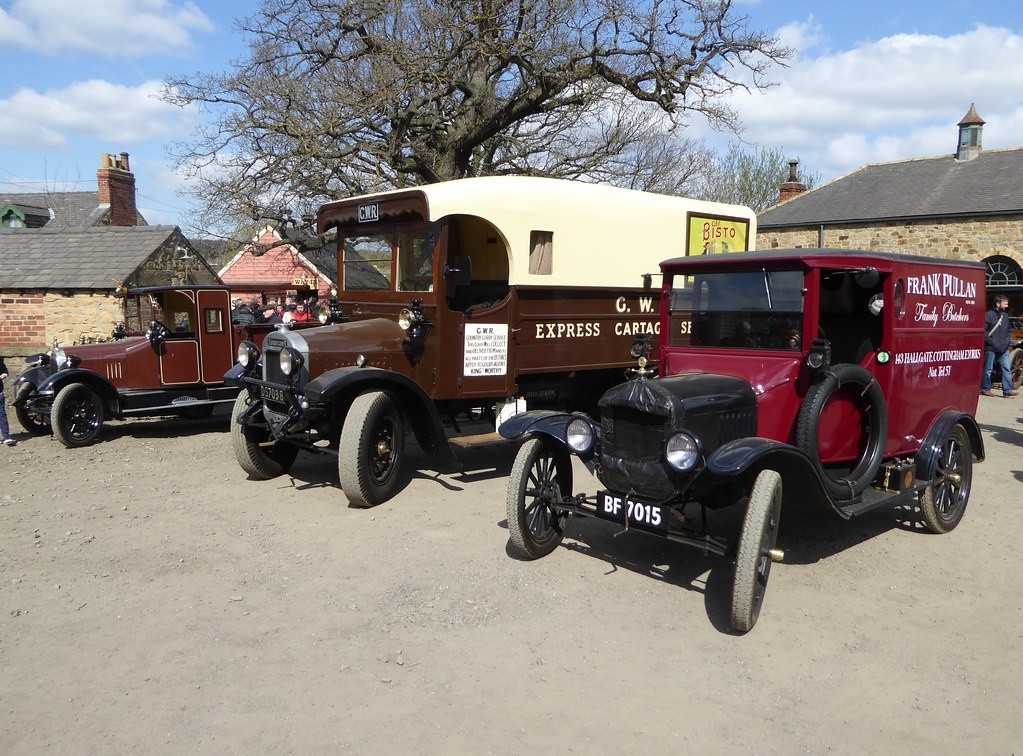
[230,175,758,507]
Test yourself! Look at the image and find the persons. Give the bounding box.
[231,297,332,334]
[981,295,1019,397]
[0,356,17,445]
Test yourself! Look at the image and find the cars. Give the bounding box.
[12,281,326,449]
[497,247,988,633]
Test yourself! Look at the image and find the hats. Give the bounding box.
[259,304,275,311]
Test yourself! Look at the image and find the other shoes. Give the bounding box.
[981,392,994,396]
[1003,390,1019,396]
[3,438,13,445]
